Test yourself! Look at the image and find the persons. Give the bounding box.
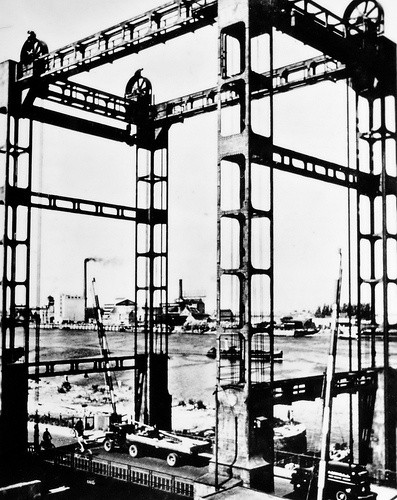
[43,428,51,443]
[74,417,84,430]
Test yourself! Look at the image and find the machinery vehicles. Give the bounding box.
[91,277,378,500]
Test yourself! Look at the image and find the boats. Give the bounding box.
[260,320,321,338]
[204,347,283,363]
[122,313,209,335]
